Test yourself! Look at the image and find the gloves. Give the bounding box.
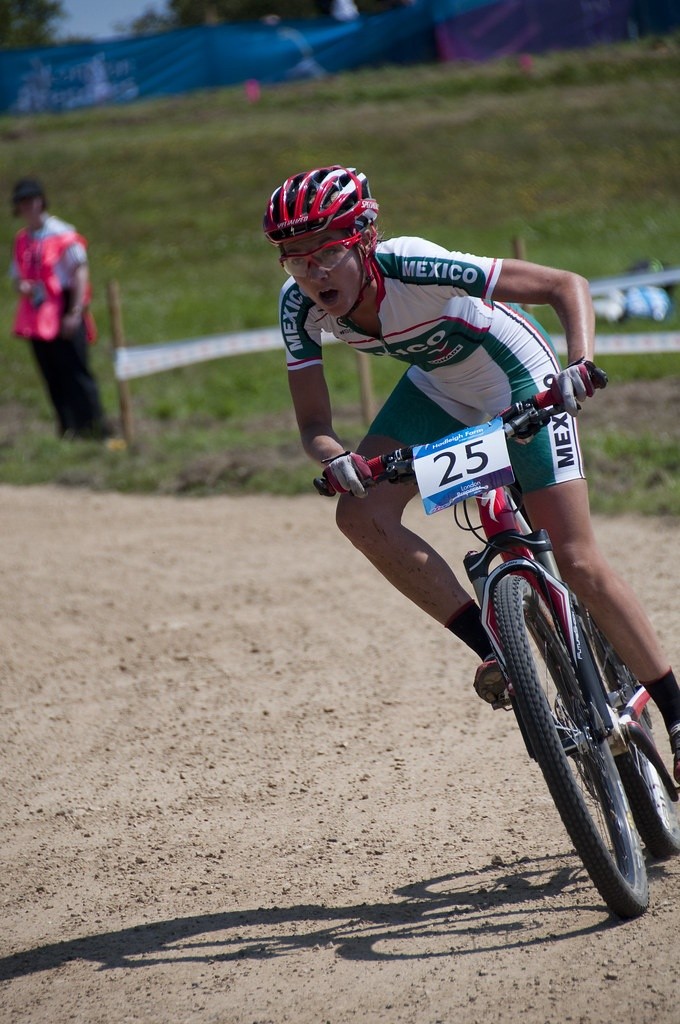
[552,356,596,417]
[322,451,373,498]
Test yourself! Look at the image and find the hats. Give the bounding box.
[12,176,42,202]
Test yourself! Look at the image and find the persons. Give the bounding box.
[263,164,679,783]
[9,178,114,446]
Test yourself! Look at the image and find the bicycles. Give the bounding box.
[312,365,680,919]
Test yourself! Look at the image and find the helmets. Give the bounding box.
[263,165,378,246]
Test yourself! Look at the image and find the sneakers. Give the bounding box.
[473,651,514,711]
[669,723,680,784]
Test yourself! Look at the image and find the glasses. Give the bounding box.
[279,227,364,277]
